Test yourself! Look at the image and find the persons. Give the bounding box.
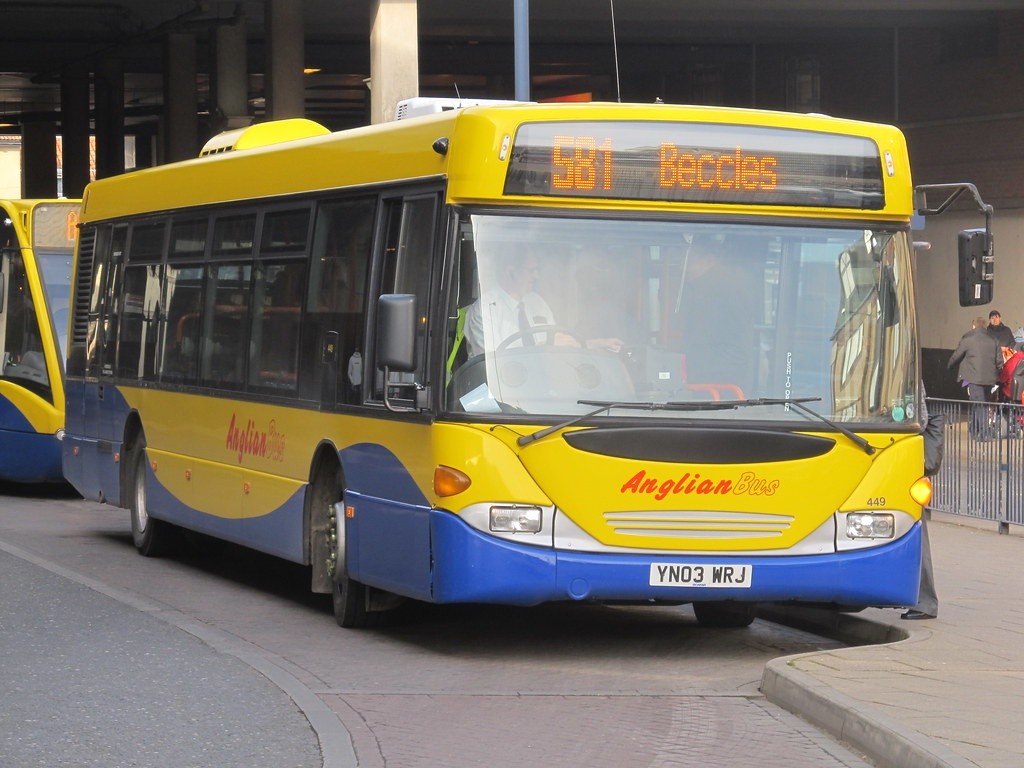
[459,237,627,362]
[992,342,1024,416]
[946,317,1004,444]
[985,310,1016,351]
[900,373,939,622]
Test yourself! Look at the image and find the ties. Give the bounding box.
[517,302,534,346]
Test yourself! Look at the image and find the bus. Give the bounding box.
[1,198,86,489]
[60,94,996,637]
